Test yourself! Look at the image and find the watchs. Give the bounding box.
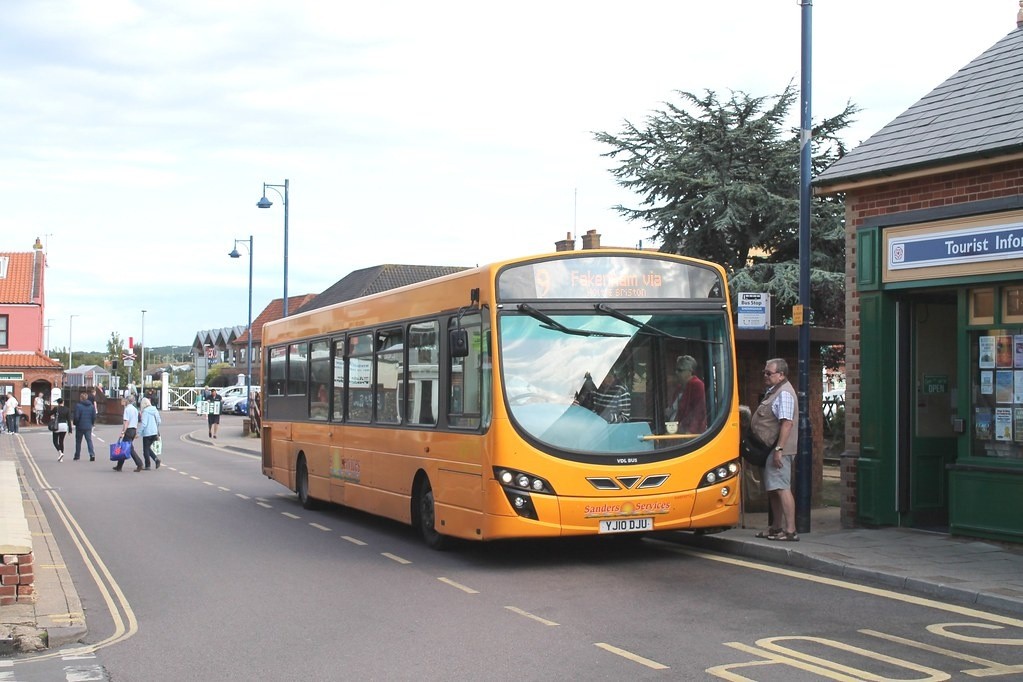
[775,446,783,451]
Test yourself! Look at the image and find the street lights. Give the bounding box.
[228,236,253,416]
[256,179,289,317]
[69,314,79,370]
[141,311,147,399]
[47,319,56,357]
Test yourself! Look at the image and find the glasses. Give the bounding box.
[762,370,779,376]
[672,368,686,374]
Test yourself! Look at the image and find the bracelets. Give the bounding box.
[121,432,125,433]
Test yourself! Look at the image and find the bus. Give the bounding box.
[259,246,742,548]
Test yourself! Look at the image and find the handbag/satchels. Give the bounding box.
[110,437,131,461]
[740,430,771,469]
[151,436,162,455]
[32,407,36,413]
[48,419,58,431]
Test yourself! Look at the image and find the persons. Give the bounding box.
[0,392,23,435]
[750,358,799,541]
[33,392,45,426]
[664,354,706,434]
[137,397,161,470]
[504,361,632,423]
[112,395,145,472]
[128,378,142,423]
[73,393,96,461]
[45,398,72,463]
[203,389,223,438]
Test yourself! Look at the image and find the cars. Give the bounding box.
[219,386,260,416]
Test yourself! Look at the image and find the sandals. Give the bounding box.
[767,529,800,541]
[755,528,782,538]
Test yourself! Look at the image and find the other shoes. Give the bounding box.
[134,466,143,472]
[57,450,64,462]
[7,431,14,435]
[209,433,217,439]
[142,467,150,470]
[36,419,44,425]
[73,457,80,460]
[90,456,95,461]
[155,459,161,469]
[112,465,121,471]
